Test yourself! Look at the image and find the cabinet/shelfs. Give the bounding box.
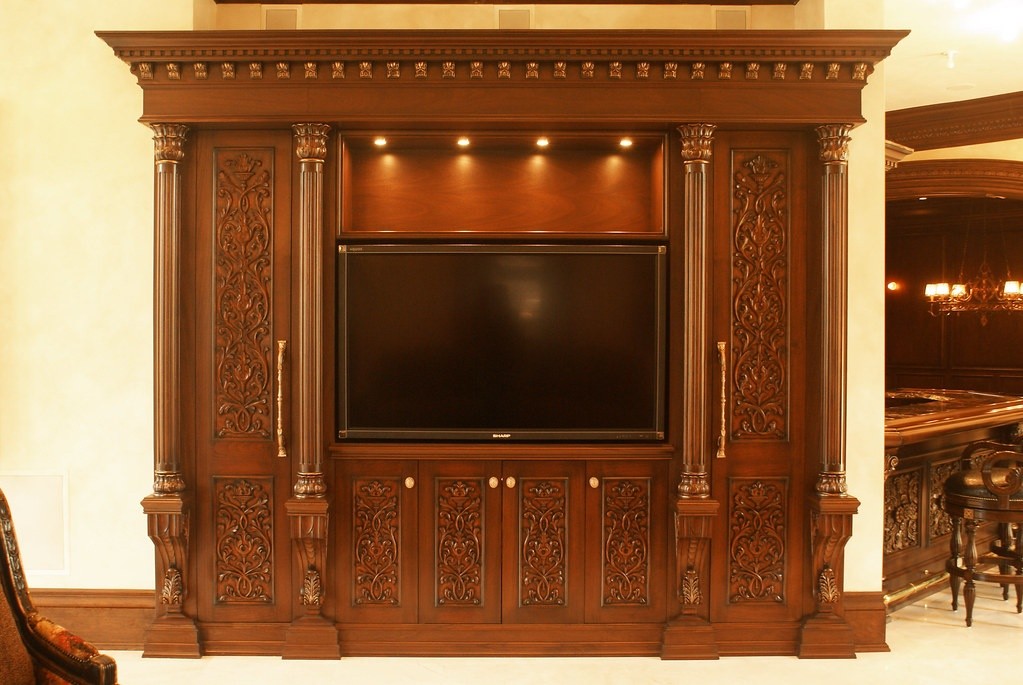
[93,27,913,663]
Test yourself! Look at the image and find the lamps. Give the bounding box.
[924,198,1023,328]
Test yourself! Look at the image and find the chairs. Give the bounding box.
[0,489,120,685]
[942,441,1023,628]
